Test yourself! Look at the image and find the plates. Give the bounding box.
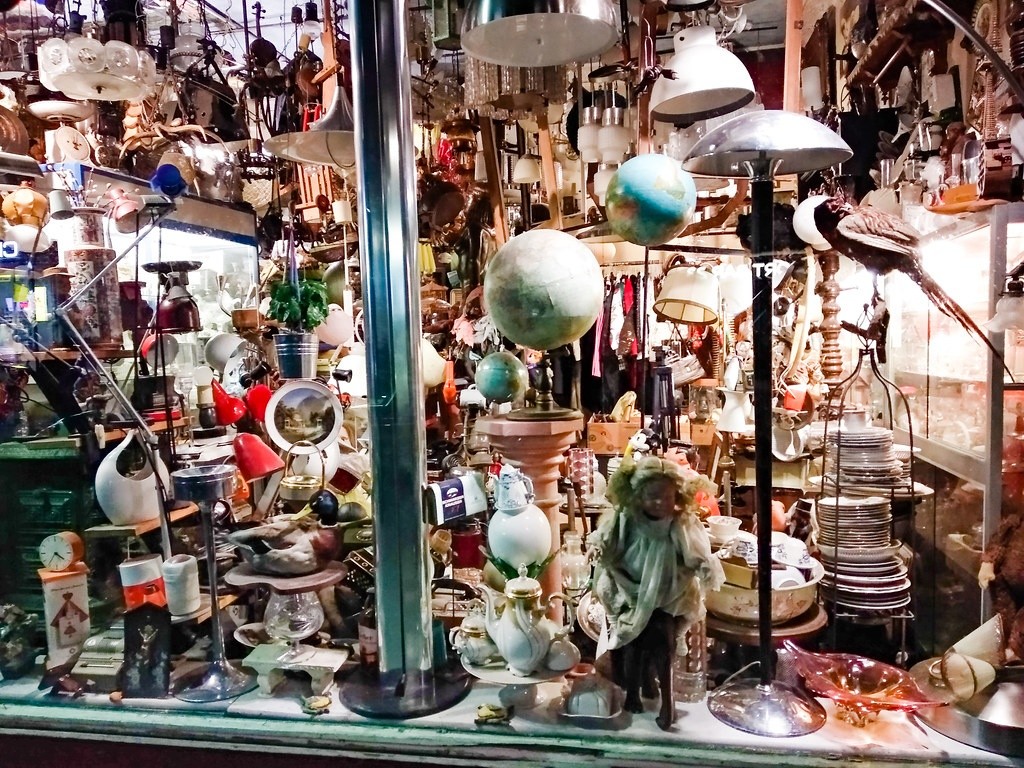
[811,495,912,610]
[771,547,818,568]
[556,698,622,719]
[717,549,760,563]
[827,426,921,485]
[705,528,757,545]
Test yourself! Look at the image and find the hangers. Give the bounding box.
[183,40,233,100]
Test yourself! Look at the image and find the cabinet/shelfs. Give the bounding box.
[0,346,253,648]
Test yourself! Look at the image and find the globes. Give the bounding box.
[605,152,697,410]
[475,353,529,405]
[485,228,605,421]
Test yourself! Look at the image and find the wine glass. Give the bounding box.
[172,343,200,451]
[263,591,324,664]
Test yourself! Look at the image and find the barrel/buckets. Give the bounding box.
[272,333,318,380]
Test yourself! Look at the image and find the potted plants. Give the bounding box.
[267,279,329,381]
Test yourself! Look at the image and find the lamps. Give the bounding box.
[642,25,756,125]
[104,275,203,430]
[433,0,476,152]
[512,132,540,184]
[465,51,569,119]
[1,181,176,234]
[36,35,157,101]
[652,262,718,325]
[681,110,854,737]
[577,63,630,207]
[233,432,286,483]
[332,200,353,355]
[459,0,619,68]
[984,260,1024,333]
[108,10,138,47]
[304,3,320,30]
[263,69,358,171]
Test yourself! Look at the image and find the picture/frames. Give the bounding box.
[265,379,344,457]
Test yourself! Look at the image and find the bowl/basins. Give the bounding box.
[233,622,331,655]
[783,638,950,728]
[15,489,73,508]
[700,555,826,624]
[170,464,238,499]
[328,638,360,657]
[771,566,807,588]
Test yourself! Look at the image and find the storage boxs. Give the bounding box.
[692,419,717,446]
[587,413,642,457]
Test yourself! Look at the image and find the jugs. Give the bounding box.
[449,609,498,665]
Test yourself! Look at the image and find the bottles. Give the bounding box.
[592,454,606,500]
[357,591,380,670]
[560,531,591,597]
[945,421,969,450]
[937,380,961,415]
[894,386,922,434]
[477,501,559,581]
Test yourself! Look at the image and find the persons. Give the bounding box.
[583,466,723,728]
[40,267,94,346]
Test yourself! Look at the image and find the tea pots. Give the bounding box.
[476,563,579,677]
[488,463,536,507]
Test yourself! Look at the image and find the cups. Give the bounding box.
[843,410,866,431]
[688,378,720,424]
[163,555,201,615]
[674,669,705,702]
[701,516,742,538]
[1004,413,1017,439]
[719,531,811,564]
[460,384,487,407]
[568,447,593,506]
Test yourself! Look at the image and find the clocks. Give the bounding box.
[38,531,85,574]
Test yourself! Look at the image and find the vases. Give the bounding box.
[63,207,125,351]
